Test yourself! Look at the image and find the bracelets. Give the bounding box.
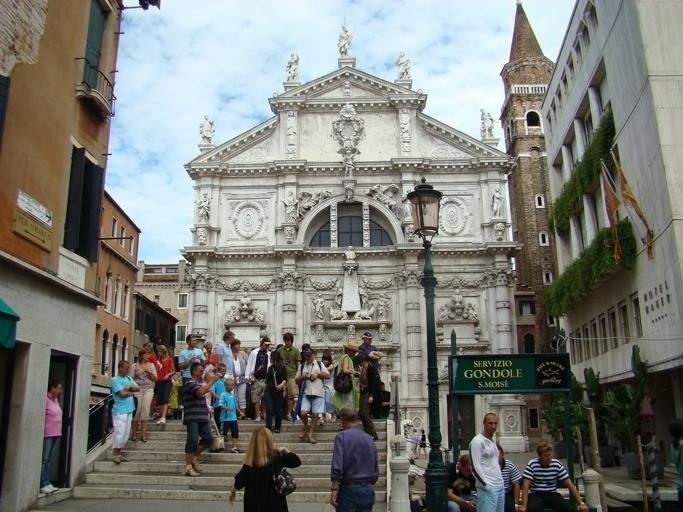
[231,491,235,493]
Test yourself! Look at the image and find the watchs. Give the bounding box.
[331,487,337,491]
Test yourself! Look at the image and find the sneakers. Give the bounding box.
[132,435,148,441]
[274,429,279,433]
[157,416,166,425]
[41,483,59,493]
[255,417,261,422]
[113,454,127,463]
[231,448,243,453]
[185,456,203,476]
[300,429,318,443]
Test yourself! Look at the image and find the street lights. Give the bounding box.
[407,176,449,511]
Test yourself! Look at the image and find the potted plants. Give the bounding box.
[543,344,664,479]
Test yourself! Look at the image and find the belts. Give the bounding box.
[346,481,373,484]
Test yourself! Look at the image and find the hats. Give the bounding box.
[362,332,374,340]
[262,337,274,345]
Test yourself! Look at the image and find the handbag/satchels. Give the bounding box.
[334,371,352,394]
[274,469,296,494]
[209,436,224,453]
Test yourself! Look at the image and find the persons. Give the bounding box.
[330,407,379,511]
[493,188,505,219]
[445,412,587,511]
[410,428,427,459]
[359,285,369,309]
[332,284,343,307]
[199,115,215,144]
[395,51,411,78]
[341,145,359,179]
[479,108,493,138]
[193,193,210,222]
[312,294,325,320]
[287,51,299,81]
[227,426,301,511]
[110,330,391,476]
[280,190,295,216]
[668,418,683,512]
[401,188,412,221]
[410,459,417,470]
[409,475,425,511]
[337,25,353,56]
[40,377,63,494]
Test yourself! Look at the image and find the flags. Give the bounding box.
[613,156,654,259]
[602,166,623,265]
